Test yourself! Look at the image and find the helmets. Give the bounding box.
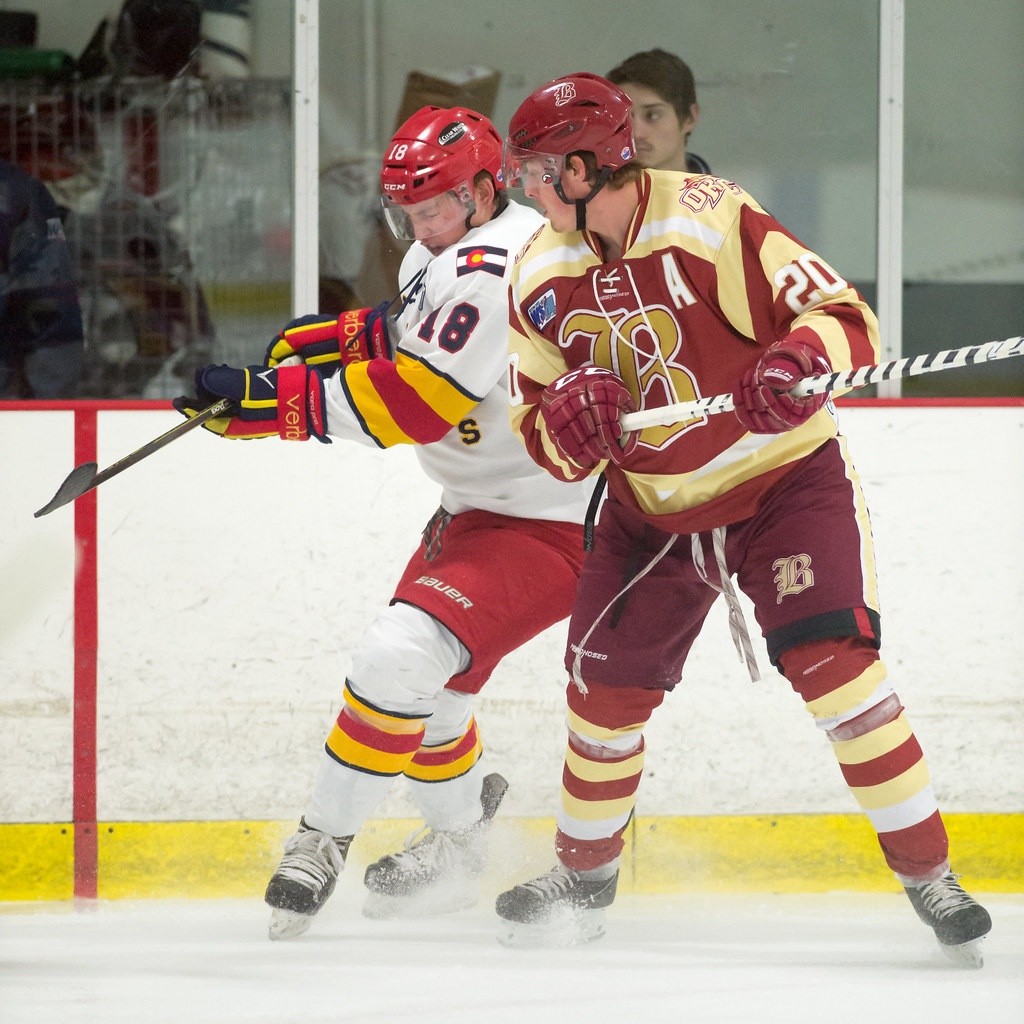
[380,106,506,241]
[501,72,637,187]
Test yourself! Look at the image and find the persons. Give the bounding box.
[491,73,997,969]
[604,50,712,174]
[11,107,217,401]
[0,162,86,401]
[170,105,609,941]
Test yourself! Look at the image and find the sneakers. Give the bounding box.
[495,860,619,945]
[896,862,991,968]
[365,773,506,922]
[265,817,354,938]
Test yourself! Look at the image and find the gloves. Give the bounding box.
[541,365,637,470]
[732,339,832,434]
[173,363,331,444]
[262,307,392,380]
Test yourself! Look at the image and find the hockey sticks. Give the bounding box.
[32,349,302,528]
[621,335,1024,434]
